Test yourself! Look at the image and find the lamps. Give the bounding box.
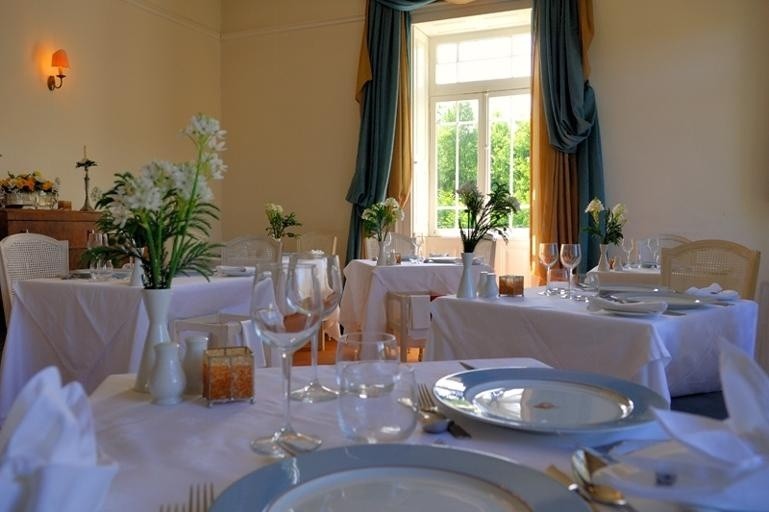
[48,49,71,91]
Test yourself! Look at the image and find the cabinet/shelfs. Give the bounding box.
[0,206,108,270]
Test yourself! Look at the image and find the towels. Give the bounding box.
[648,333,769,469]
[0,366,119,512]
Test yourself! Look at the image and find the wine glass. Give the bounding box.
[411,232,424,261]
[250,250,420,457]
[383,231,391,251]
[86,227,109,274]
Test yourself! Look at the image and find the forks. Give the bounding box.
[189,481,214,512]
[160,501,185,511]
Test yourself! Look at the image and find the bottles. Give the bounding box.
[477,272,488,297]
[486,273,498,299]
[148,342,187,406]
[181,336,208,395]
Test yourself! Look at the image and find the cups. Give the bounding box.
[537,237,659,299]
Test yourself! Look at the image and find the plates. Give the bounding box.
[208,443,590,512]
[433,367,669,434]
[599,284,705,316]
[428,257,458,263]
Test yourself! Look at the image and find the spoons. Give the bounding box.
[569,446,635,512]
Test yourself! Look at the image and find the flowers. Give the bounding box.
[455,181,521,254]
[581,197,628,248]
[362,198,404,241]
[78,112,229,289]
[263,202,303,239]
[0,169,58,194]
